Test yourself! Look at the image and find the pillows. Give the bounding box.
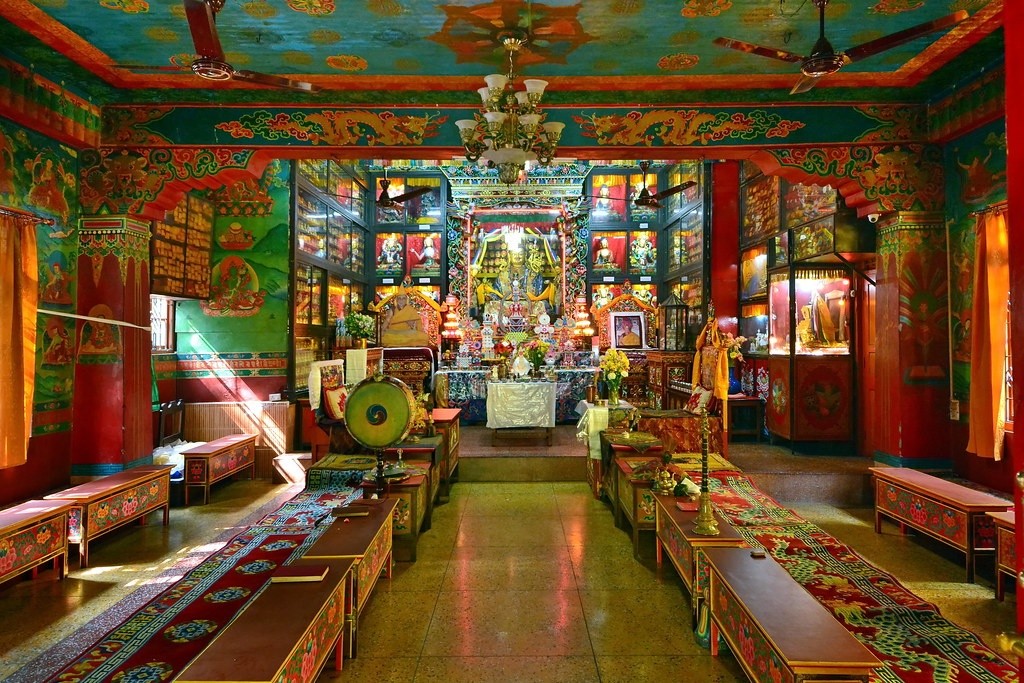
[683,383,714,415]
[322,385,348,419]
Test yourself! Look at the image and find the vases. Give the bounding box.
[497,361,507,381]
[727,368,741,394]
[352,339,367,349]
[531,366,540,379]
[609,388,620,403]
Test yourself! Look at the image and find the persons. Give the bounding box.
[410,237,441,276]
[376,233,403,276]
[618,318,640,346]
[633,289,652,306]
[483,244,558,325]
[592,184,623,223]
[381,282,429,347]
[629,234,657,275]
[795,305,817,348]
[630,182,657,222]
[593,236,621,274]
[591,284,613,309]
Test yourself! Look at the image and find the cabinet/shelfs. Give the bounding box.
[292,161,447,398]
[586,160,710,410]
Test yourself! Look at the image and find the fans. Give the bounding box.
[712,1,969,95]
[585,160,697,206]
[108,0,323,95]
[319,167,432,211]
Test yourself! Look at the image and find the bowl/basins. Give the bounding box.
[501,375,555,383]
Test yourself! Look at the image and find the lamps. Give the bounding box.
[455,29,566,185]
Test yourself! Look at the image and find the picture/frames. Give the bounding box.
[610,312,645,350]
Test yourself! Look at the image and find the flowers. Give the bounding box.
[521,340,557,370]
[599,348,630,390]
[493,340,514,360]
[727,333,761,366]
[344,311,376,339]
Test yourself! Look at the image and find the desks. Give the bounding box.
[667,388,764,444]
[488,380,557,447]
[346,347,384,393]
[574,398,1015,683]
[0,408,461,683]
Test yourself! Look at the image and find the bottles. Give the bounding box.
[585,382,595,403]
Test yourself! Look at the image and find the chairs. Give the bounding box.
[308,360,358,455]
